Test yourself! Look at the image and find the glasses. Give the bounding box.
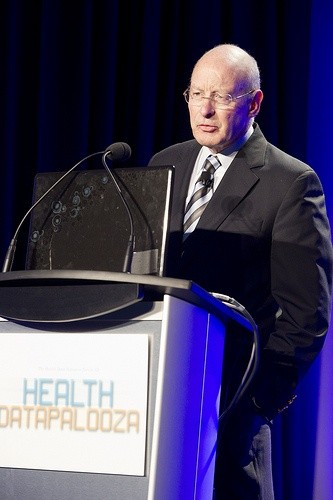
[182,85,256,106]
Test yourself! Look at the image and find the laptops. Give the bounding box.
[27,166,175,276]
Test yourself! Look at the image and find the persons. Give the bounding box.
[140,44,333,500]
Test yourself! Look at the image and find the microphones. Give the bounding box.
[206,179,213,186]
[3,143,136,273]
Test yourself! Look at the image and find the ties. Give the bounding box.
[181,154,222,242]
